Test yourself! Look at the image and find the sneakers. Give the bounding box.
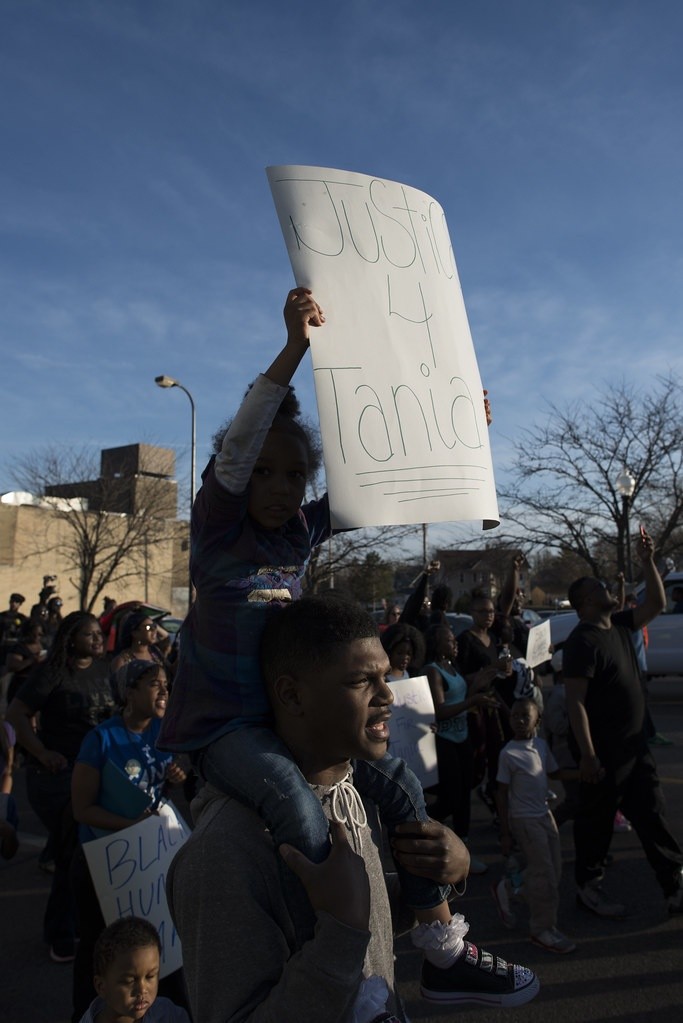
[667,890,683,916]
[420,943,539,1008]
[529,926,578,954]
[575,885,627,919]
[489,881,516,931]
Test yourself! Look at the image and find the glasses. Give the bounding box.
[585,582,611,596]
[471,609,496,616]
[137,623,157,631]
[35,611,48,615]
[50,603,62,607]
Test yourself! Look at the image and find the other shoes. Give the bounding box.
[50,941,75,962]
[38,859,55,873]
[470,858,489,873]
[648,733,674,747]
[614,822,631,833]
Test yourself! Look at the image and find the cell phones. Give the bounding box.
[639,525,646,548]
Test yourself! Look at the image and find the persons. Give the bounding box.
[76,916,190,1023]
[154,288,541,1023]
[0,530,683,1023]
